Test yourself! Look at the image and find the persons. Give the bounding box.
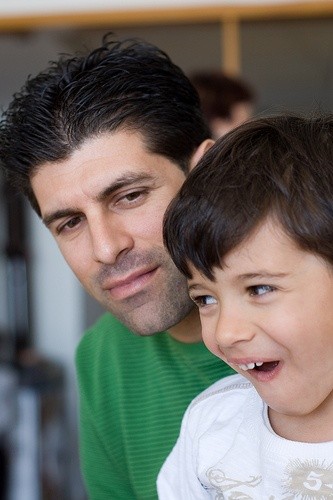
[0,33,238,500]
[154,112,332,500]
[191,70,259,143]
